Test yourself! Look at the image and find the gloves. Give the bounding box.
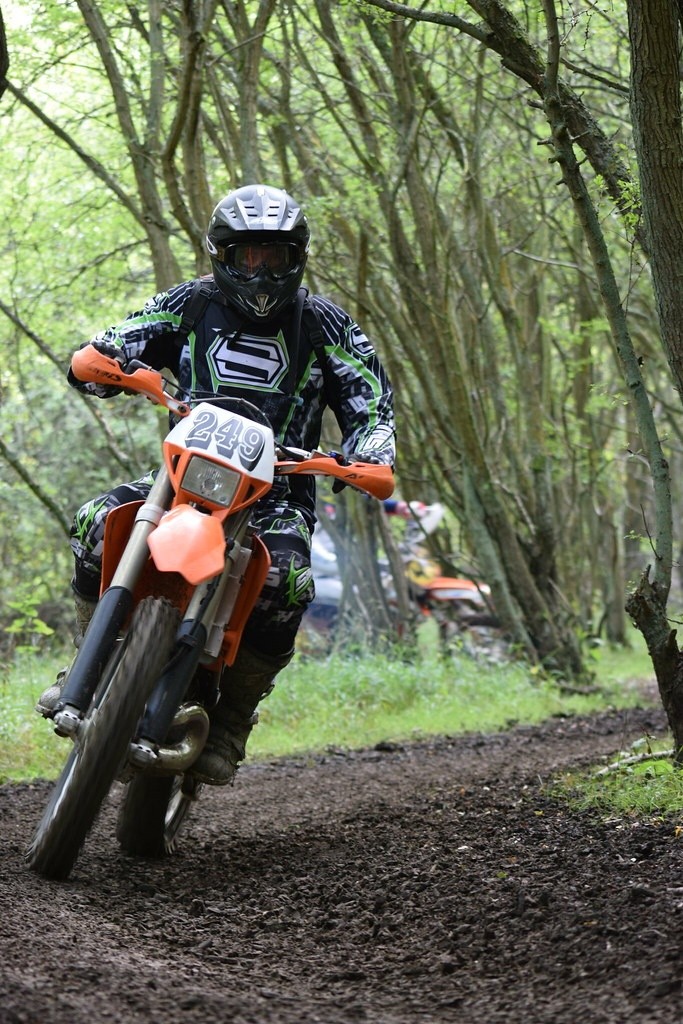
[81,341,126,373]
[332,454,382,494]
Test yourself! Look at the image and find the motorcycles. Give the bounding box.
[300,503,519,675]
[20,345,396,887]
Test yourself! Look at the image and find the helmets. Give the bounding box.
[205,185,311,322]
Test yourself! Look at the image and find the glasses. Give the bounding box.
[222,240,302,279]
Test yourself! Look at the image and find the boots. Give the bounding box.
[194,670,279,786]
[34,594,98,716]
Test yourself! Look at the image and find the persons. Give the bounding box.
[38,182,398,785]
